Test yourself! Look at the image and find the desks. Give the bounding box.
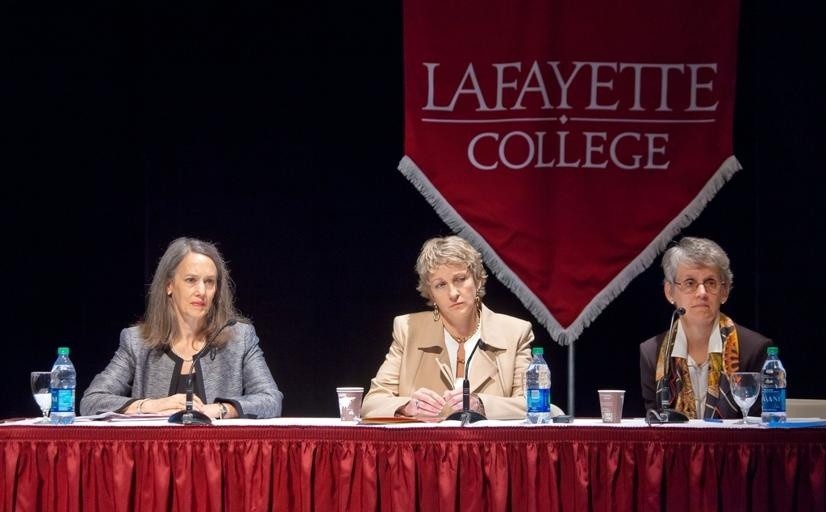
[0,417,826,512]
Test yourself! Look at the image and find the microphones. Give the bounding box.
[645,306,689,422]
[170,319,238,422]
[448,339,485,422]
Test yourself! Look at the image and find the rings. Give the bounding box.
[418,399,421,408]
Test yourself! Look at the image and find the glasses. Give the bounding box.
[674,277,726,295]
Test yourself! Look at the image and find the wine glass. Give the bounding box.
[29,371,53,425]
[729,372,762,426]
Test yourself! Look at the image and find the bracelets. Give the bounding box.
[215,399,227,418]
[134,397,149,413]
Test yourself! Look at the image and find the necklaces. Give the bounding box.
[458,357,469,365]
[172,337,207,361]
[440,312,480,342]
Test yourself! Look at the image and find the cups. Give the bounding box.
[597,389,626,424]
[336,387,364,422]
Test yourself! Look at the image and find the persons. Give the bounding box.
[78,236,283,417]
[360,235,536,419]
[641,238,775,419]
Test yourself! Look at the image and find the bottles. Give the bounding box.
[761,348,787,427]
[48,348,75,424]
[523,348,552,425]
